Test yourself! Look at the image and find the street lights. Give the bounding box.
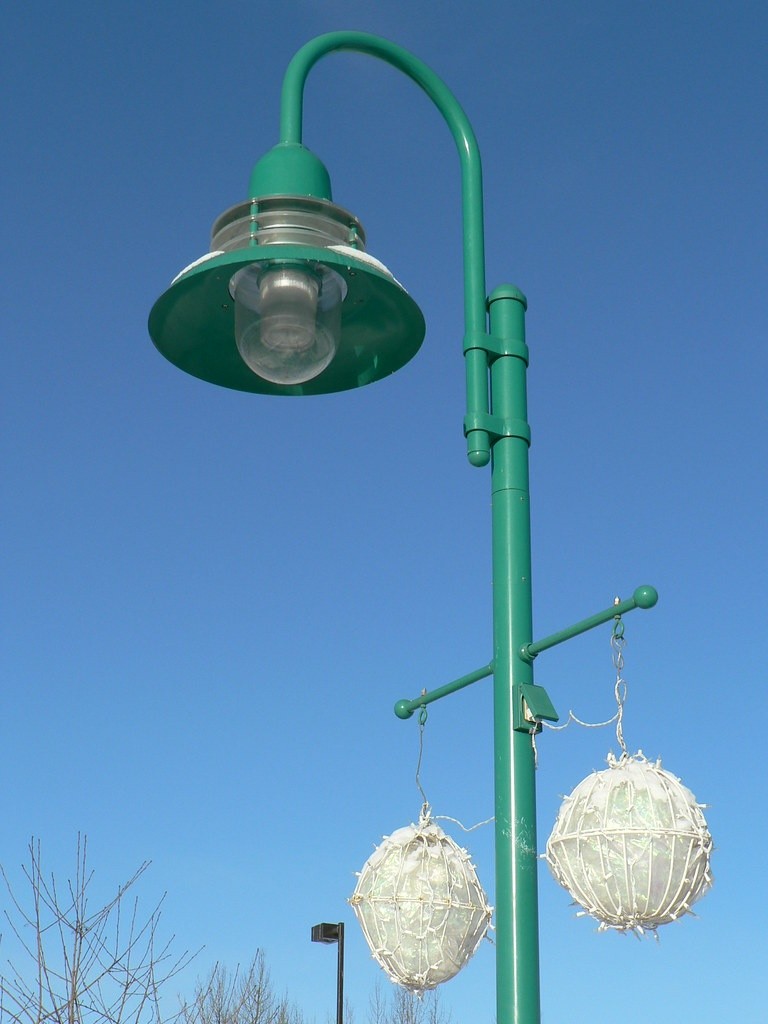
[309,922,346,1024]
[147,31,714,1024]
